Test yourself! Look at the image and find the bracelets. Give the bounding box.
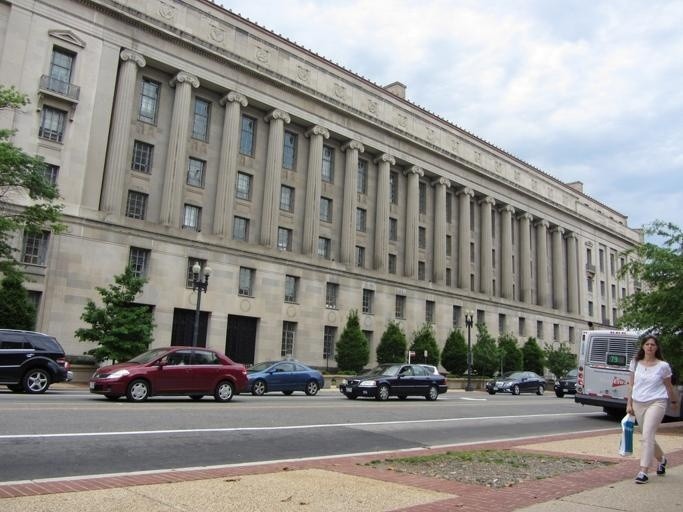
[669,399,677,404]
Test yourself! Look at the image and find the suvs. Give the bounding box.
[0,327,74,395]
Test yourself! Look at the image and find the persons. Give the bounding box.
[622,334,678,484]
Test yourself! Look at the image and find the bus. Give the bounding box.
[571,326,683,423]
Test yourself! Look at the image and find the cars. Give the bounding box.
[339,363,449,403]
[239,357,325,398]
[88,346,250,408]
[483,369,547,397]
[553,368,577,398]
[416,364,442,377]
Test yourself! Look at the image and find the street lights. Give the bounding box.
[463,308,476,392]
[190,261,211,345]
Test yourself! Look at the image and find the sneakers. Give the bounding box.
[656,459,667,474]
[634,471,648,484]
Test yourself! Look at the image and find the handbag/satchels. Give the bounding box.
[619,413,635,457]
